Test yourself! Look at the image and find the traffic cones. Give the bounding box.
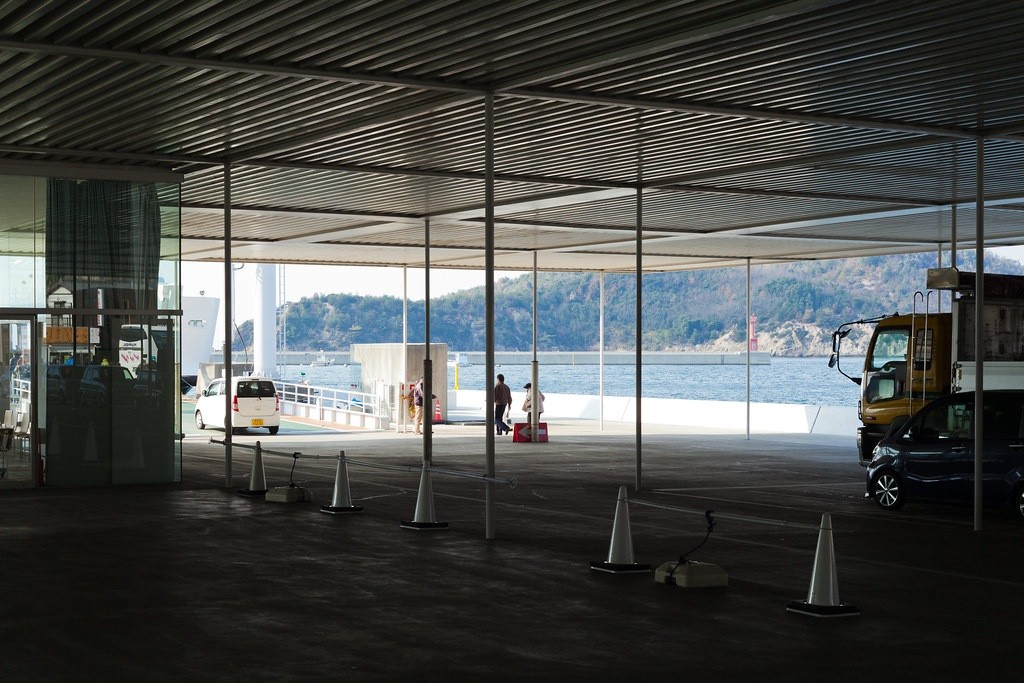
[785,514,862,618]
[431,399,446,425]
[398,459,450,533]
[237,442,271,497]
[318,452,363,516]
[590,487,652,575]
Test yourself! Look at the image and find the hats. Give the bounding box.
[523,383,532,389]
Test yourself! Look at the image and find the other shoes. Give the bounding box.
[506,428,510,435]
[415,431,423,434]
[432,431,434,433]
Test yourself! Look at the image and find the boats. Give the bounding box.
[312,353,336,367]
[447,359,473,368]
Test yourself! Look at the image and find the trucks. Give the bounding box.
[829,267,1024,466]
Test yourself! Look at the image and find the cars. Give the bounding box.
[865,388,1024,519]
[133,369,168,409]
[81,366,139,404]
[47,365,112,405]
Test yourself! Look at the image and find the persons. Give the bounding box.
[413,376,437,434]
[494,374,512,435]
[522,383,545,423]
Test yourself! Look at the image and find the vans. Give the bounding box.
[194,377,280,434]
